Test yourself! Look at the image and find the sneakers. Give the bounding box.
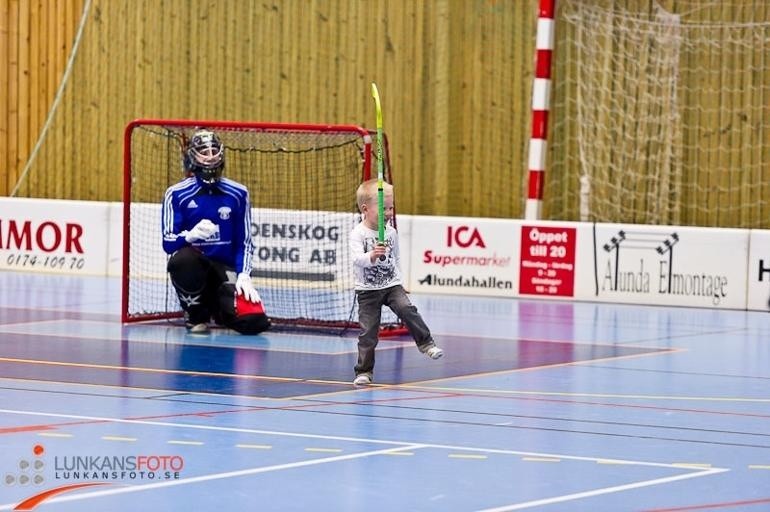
[422,345,445,360]
[353,371,374,386]
[190,323,211,334]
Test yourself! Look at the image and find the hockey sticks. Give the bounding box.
[371,83,385,260]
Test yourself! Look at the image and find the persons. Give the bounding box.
[161,130,272,334]
[347,177,443,386]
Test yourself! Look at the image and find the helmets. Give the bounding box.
[185,129,224,159]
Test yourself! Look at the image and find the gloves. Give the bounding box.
[185,218,217,244]
[235,272,262,304]
